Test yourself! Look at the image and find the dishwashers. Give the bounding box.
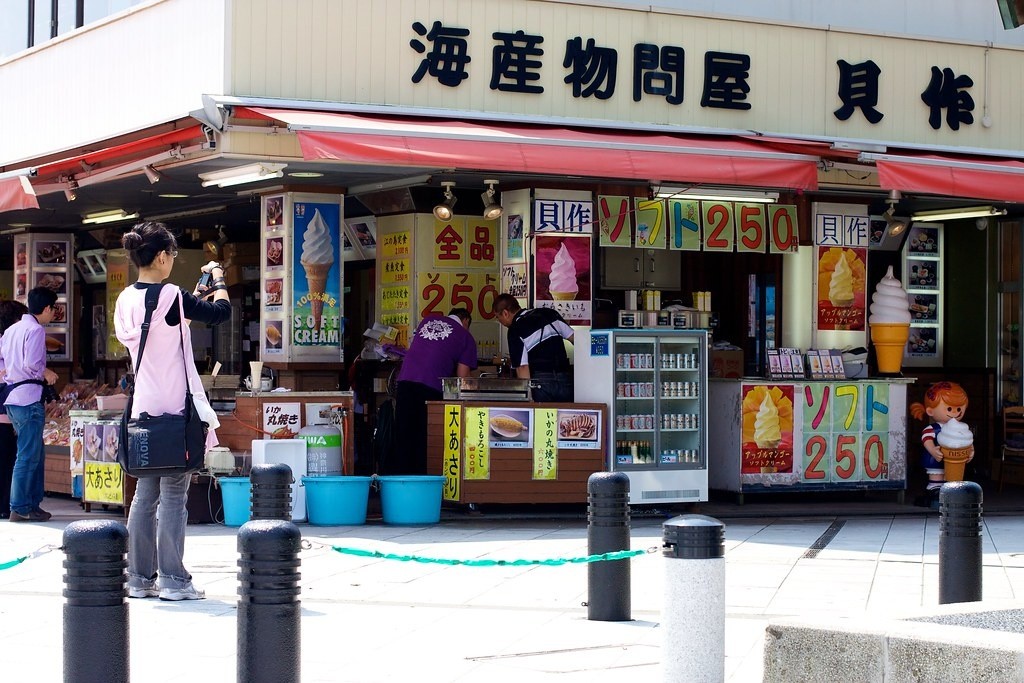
[922,262,929,269]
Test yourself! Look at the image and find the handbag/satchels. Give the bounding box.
[118,393,210,477]
[0,378,43,414]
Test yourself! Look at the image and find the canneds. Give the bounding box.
[615,414,654,429]
[616,382,653,397]
[616,353,653,369]
[663,448,697,463]
[659,353,698,430]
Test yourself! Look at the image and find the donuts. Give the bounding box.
[912,278,933,352]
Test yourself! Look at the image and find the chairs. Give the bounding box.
[999,406,1024,495]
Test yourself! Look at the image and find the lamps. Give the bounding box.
[197,162,287,188]
[143,164,161,184]
[883,208,906,238]
[433,182,458,222]
[348,174,432,195]
[81,208,142,224]
[909,205,1008,222]
[650,185,779,203]
[480,179,504,220]
[62,185,77,201]
[207,226,227,254]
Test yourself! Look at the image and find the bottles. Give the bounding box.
[664,450,696,462]
[617,440,650,463]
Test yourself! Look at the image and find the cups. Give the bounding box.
[920,329,929,341]
[915,295,922,305]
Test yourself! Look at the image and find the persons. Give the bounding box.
[385,307,478,476]
[113,220,231,601]
[0,285,59,523]
[491,293,574,403]
[910,381,974,509]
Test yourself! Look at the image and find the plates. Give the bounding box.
[490,414,521,437]
[266,206,280,345]
[36,247,65,352]
[558,413,597,441]
[85,432,120,460]
[909,333,927,346]
[909,304,928,313]
[918,269,928,277]
[871,231,882,242]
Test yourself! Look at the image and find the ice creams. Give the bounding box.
[937,417,974,481]
[300,209,334,331]
[869,266,912,373]
[548,242,578,302]
[771,356,841,374]
[753,392,782,473]
[829,252,854,330]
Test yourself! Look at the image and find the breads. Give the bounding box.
[266,326,280,340]
[45,336,63,352]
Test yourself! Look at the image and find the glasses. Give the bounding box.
[157,249,178,258]
[50,305,58,311]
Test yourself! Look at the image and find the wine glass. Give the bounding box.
[929,304,935,315]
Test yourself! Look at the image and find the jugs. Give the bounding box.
[244,365,273,391]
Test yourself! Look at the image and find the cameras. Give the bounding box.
[196,270,213,292]
[43,378,61,404]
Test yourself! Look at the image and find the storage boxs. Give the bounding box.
[252,439,309,522]
[203,243,259,287]
[95,396,128,411]
[709,350,744,379]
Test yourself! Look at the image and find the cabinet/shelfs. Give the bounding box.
[599,241,681,291]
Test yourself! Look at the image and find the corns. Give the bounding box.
[490,416,528,432]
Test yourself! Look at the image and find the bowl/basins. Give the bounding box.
[912,344,928,352]
[916,313,927,318]
[920,273,934,284]
[911,233,937,250]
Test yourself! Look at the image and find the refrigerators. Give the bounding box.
[574,329,708,504]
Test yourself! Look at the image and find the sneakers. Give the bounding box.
[127,584,162,598]
[159,582,206,601]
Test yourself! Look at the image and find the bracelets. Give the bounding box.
[209,262,226,276]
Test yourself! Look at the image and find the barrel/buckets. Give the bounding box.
[301,476,374,524]
[218,477,254,526]
[374,476,446,524]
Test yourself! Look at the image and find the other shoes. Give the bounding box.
[0,508,10,519]
[9,506,51,522]
